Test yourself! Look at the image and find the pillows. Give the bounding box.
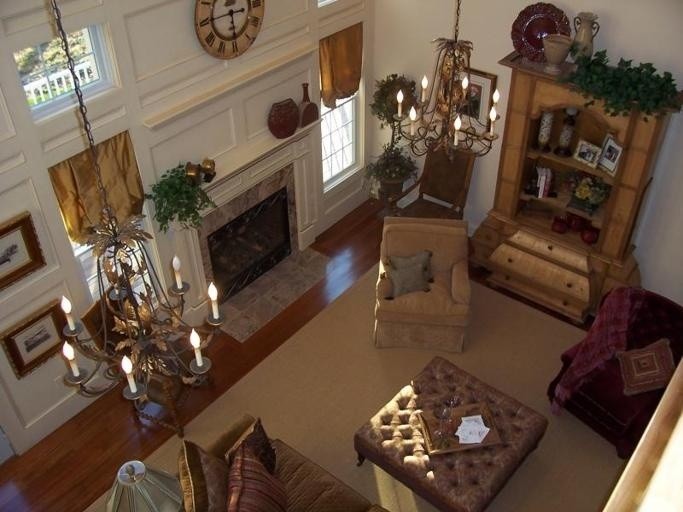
[228,440,286,512]
[225,418,276,472]
[617,338,675,396]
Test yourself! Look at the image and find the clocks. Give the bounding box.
[195,0,265,59]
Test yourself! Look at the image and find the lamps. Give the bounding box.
[45,0,226,413]
[397,1,500,157]
[105,460,183,512]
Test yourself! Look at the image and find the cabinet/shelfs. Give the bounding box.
[470,50,683,327]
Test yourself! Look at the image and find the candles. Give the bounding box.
[490,106,497,136]
[410,106,416,135]
[492,87,500,106]
[396,89,403,118]
[462,76,469,100]
[62,341,80,377]
[421,75,428,103]
[208,281,219,319]
[121,355,137,394]
[172,255,183,289]
[454,115,461,145]
[60,295,76,331]
[189,327,203,367]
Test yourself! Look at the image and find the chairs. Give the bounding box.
[374,141,475,262]
[81,274,212,439]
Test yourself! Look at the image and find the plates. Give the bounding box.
[417,402,504,457]
[510,1,571,64]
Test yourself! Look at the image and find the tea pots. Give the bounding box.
[580,229,597,244]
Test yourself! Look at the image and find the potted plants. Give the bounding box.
[365,74,419,217]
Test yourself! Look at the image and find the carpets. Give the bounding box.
[82,260,631,512]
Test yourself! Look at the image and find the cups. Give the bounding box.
[432,397,451,439]
[541,33,573,76]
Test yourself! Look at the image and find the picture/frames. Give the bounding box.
[459,68,498,139]
[598,132,627,179]
[0,299,76,380]
[573,140,602,169]
[0,211,47,292]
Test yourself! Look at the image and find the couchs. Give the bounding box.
[547,286,683,459]
[374,216,471,353]
[180,413,391,512]
